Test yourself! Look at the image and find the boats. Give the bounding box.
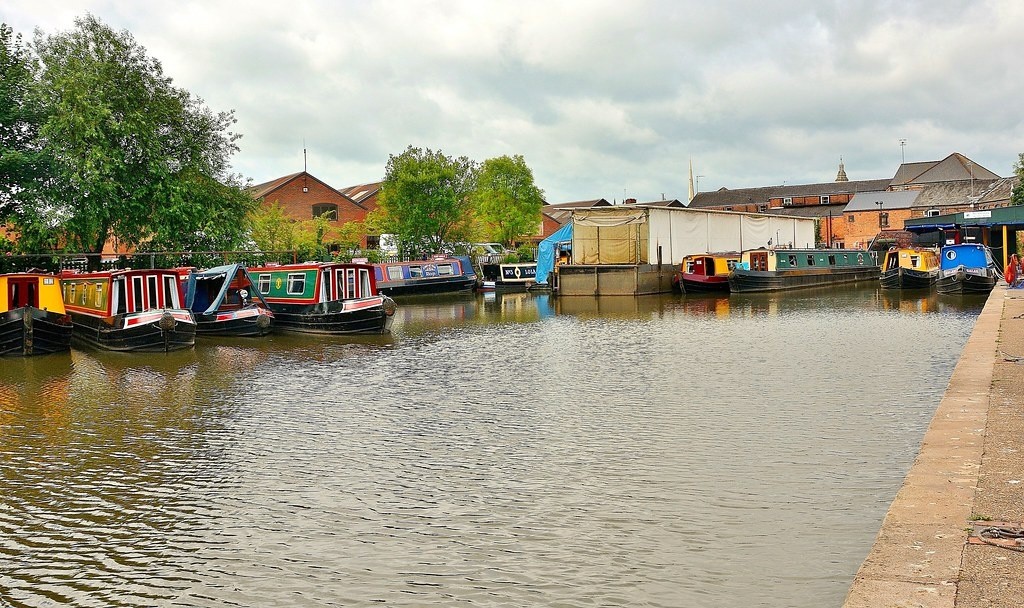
[59,267,197,350]
[936,243,997,296]
[674,229,882,293]
[880,245,940,288]
[0,268,73,356]
[245,261,397,334]
[176,263,276,336]
[373,255,477,294]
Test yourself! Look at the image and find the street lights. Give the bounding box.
[697,175,705,195]
[967,160,974,210]
[898,138,907,191]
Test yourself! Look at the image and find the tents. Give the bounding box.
[903,205,1024,274]
[553,205,821,265]
